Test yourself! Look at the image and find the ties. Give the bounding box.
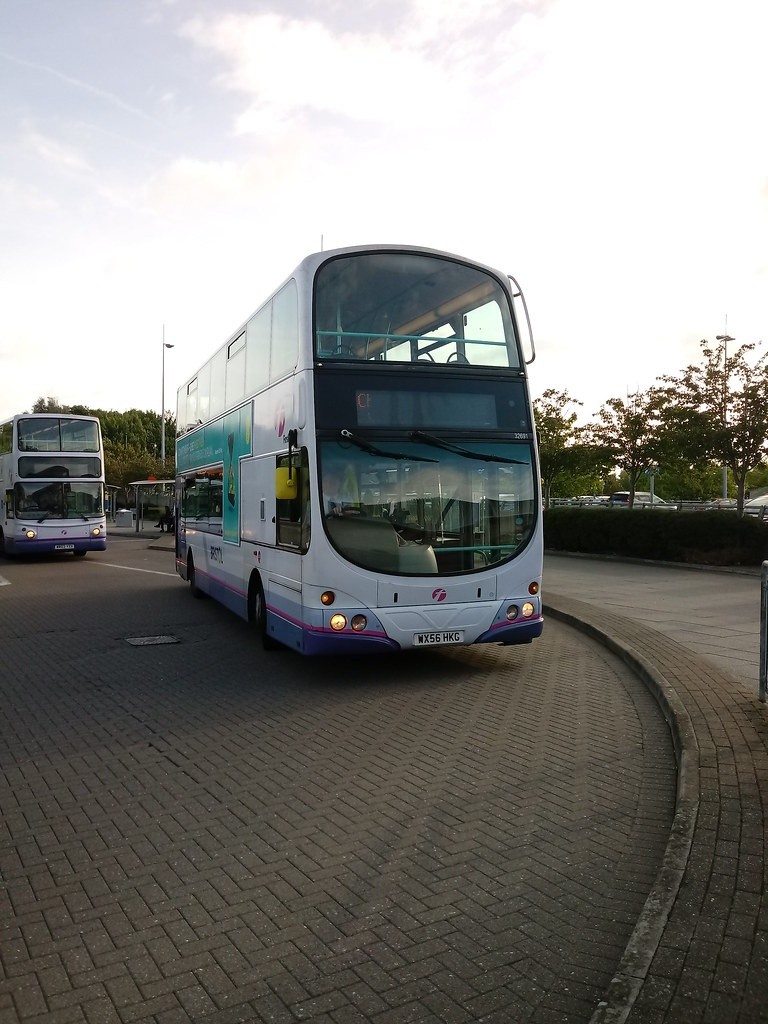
[330,499,336,509]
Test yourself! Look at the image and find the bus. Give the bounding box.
[172,244,546,667]
[0,412,110,557]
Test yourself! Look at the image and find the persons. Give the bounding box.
[19,492,39,510]
[154,506,172,532]
[302,473,343,525]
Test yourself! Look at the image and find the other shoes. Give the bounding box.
[160,530,164,532]
[154,525,160,527]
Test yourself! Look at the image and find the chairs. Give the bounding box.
[329,343,469,365]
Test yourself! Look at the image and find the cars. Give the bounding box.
[693,493,767,523]
[542,489,678,510]
